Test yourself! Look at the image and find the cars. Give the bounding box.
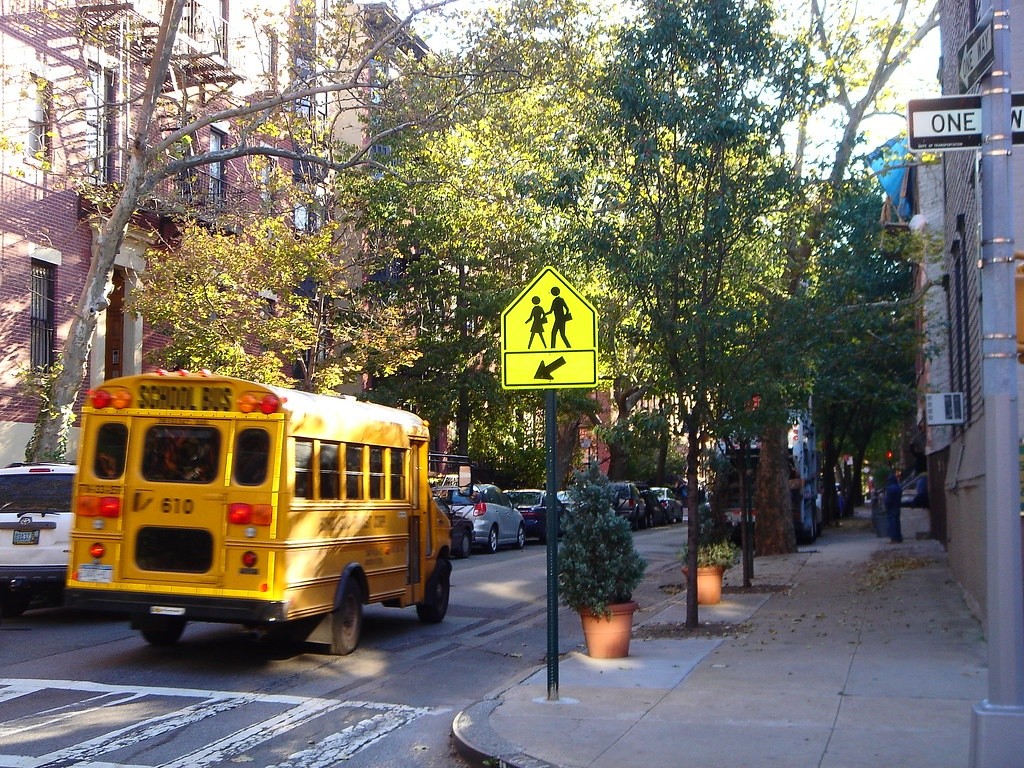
[501,489,570,545]
[431,495,475,559]
[556,490,589,521]
[430,482,527,555]
[648,487,685,524]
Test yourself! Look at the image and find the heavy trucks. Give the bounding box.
[706,407,822,546]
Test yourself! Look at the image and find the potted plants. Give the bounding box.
[557,452,649,659]
[680,503,741,605]
[872,462,894,537]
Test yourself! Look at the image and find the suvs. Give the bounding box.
[0,461,79,610]
[634,483,669,529]
[605,482,649,531]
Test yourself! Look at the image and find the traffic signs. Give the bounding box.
[908,91,1024,153]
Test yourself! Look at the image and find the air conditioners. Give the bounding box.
[923,392,963,425]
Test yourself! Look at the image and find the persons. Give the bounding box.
[884,473,903,543]
[909,441,927,494]
[868,480,877,509]
[837,486,844,512]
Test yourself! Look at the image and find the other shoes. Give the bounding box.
[888,539,901,544]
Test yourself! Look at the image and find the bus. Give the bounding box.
[62,367,484,656]
